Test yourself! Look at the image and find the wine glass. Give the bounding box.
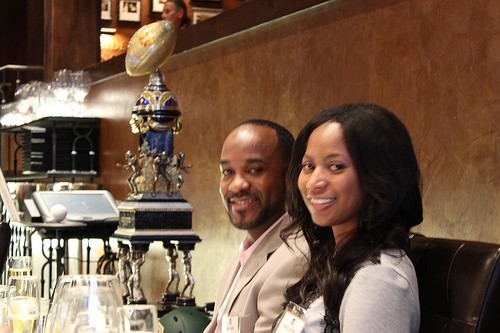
[14,81,50,114]
[52,68,69,112]
[70,69,92,113]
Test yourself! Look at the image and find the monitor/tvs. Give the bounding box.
[32,189,119,222]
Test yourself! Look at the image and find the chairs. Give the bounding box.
[406,230,500,333]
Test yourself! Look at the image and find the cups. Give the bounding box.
[6,256,33,279]
[6,277,41,333]
[123,303,156,333]
[43,273,130,332]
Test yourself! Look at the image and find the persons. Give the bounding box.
[115,147,197,307]
[269,102,425,333]
[161,0,191,31]
[201,117,312,333]
